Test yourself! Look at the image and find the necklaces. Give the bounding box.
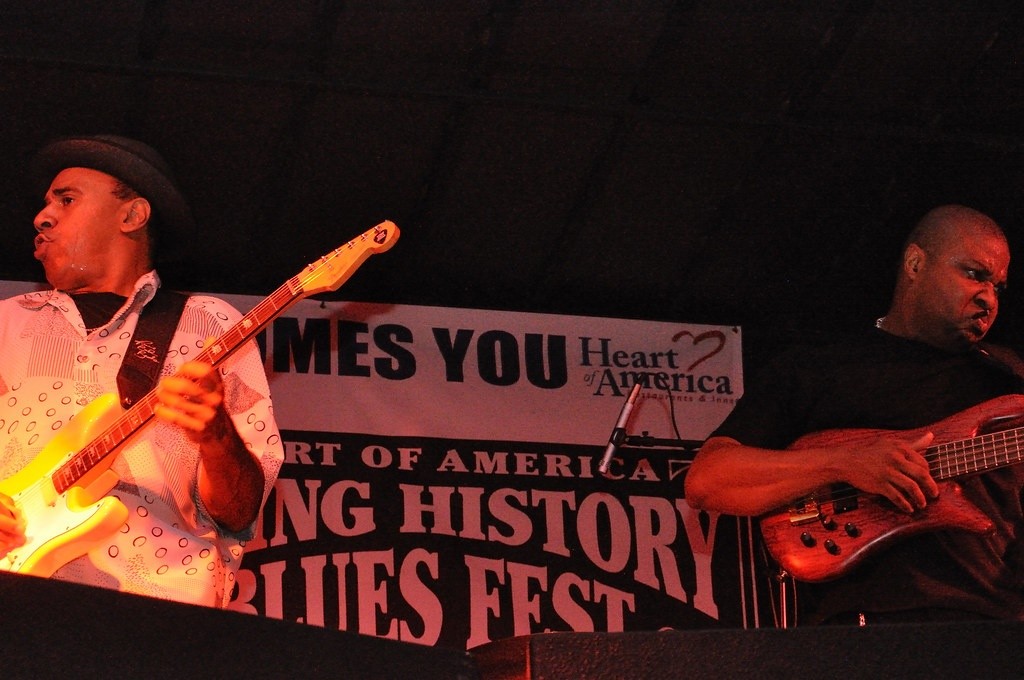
[84,326,100,332]
[875,315,888,329]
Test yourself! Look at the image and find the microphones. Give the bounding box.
[598,374,648,474]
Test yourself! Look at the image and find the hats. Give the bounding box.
[20,131,197,236]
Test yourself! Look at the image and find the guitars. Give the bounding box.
[760,392,1024,582]
[2,215,403,578]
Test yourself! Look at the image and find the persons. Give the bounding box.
[685,204,1024,628]
[0,134,284,611]
